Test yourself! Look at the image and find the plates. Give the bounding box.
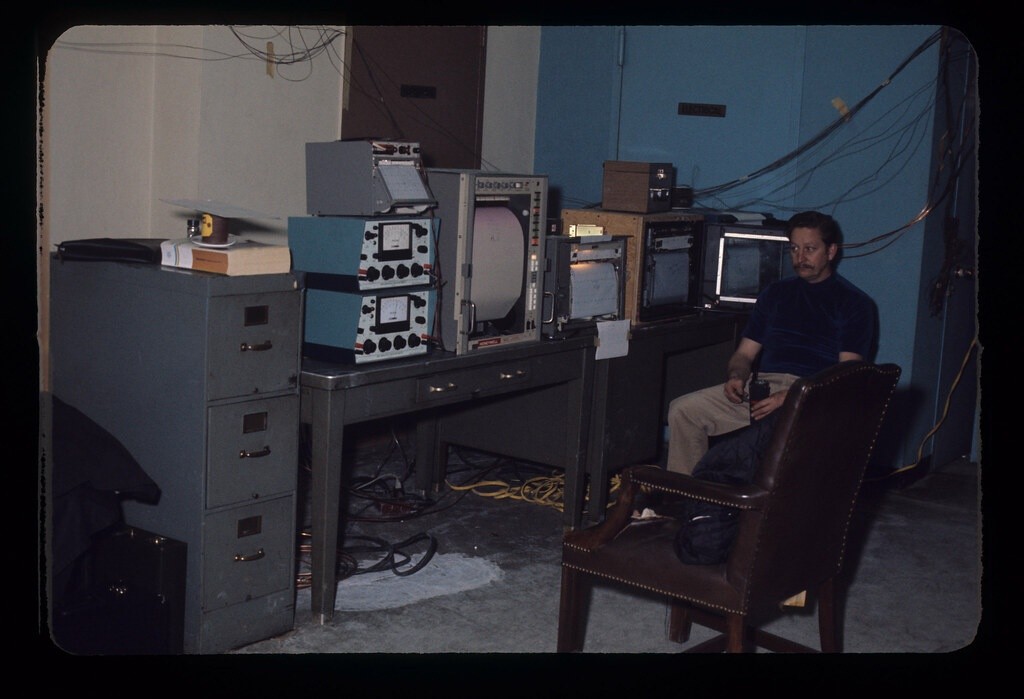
[190,234,236,248]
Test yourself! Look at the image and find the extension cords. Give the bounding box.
[373,492,424,515]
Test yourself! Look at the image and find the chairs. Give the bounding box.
[557,362,903,655]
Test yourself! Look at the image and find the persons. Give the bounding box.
[659,211,879,521]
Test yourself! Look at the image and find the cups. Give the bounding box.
[201,211,228,244]
[749,379,770,425]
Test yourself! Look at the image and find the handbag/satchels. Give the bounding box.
[675,506,739,566]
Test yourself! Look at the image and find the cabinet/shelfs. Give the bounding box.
[49,253,303,648]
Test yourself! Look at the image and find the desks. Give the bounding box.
[296,322,601,627]
[430,301,757,525]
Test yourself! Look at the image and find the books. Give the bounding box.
[161,233,291,276]
